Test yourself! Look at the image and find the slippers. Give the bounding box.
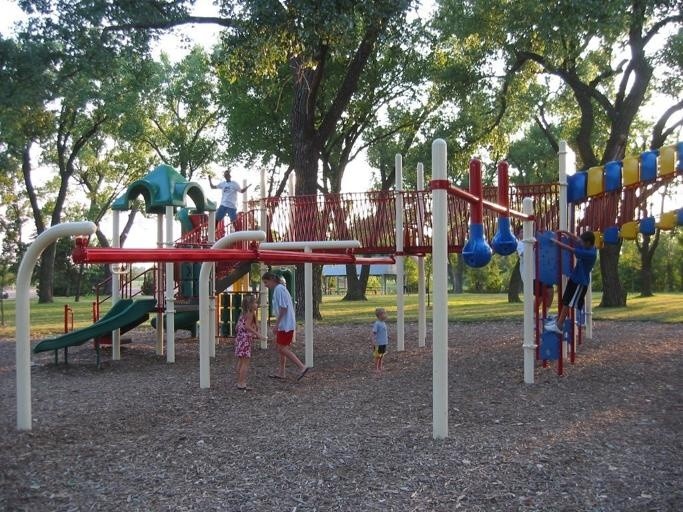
[270,374,285,379]
[237,385,252,390]
[296,367,307,380]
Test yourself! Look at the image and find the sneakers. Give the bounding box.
[544,318,563,335]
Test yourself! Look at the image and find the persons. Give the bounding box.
[370,307,391,371]
[514,225,554,321]
[234,293,268,390]
[259,271,308,380]
[542,227,598,335]
[207,170,251,233]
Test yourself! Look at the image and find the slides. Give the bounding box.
[150,260,254,339]
[33,298,156,354]
